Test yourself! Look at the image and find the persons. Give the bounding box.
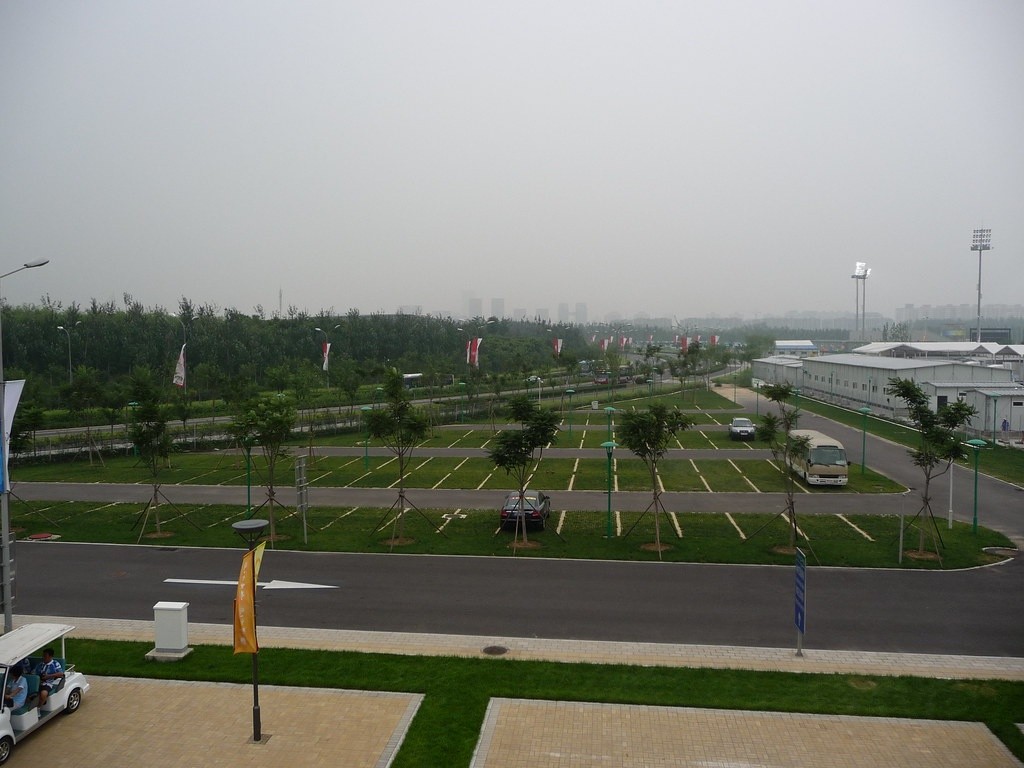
[2,657,30,712]
[29,649,64,717]
[1001,418,1010,441]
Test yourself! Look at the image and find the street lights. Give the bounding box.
[600,441,622,539]
[646,380,653,401]
[457,382,468,425]
[600,322,633,348]
[315,324,342,390]
[793,389,802,430]
[54,319,83,386]
[603,406,618,443]
[965,439,987,534]
[456,318,496,400]
[987,390,1001,448]
[859,405,873,473]
[754,380,760,417]
[168,312,200,405]
[564,389,577,444]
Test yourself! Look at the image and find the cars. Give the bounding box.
[499,489,552,531]
[729,416,759,442]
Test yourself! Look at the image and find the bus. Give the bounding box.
[399,373,455,392]
[594,364,633,384]
[783,429,852,488]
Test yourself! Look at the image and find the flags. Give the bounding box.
[232,549,258,655]
[173,335,721,385]
[251,542,266,596]
[4,380,26,492]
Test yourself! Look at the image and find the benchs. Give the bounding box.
[0,656,65,730]
